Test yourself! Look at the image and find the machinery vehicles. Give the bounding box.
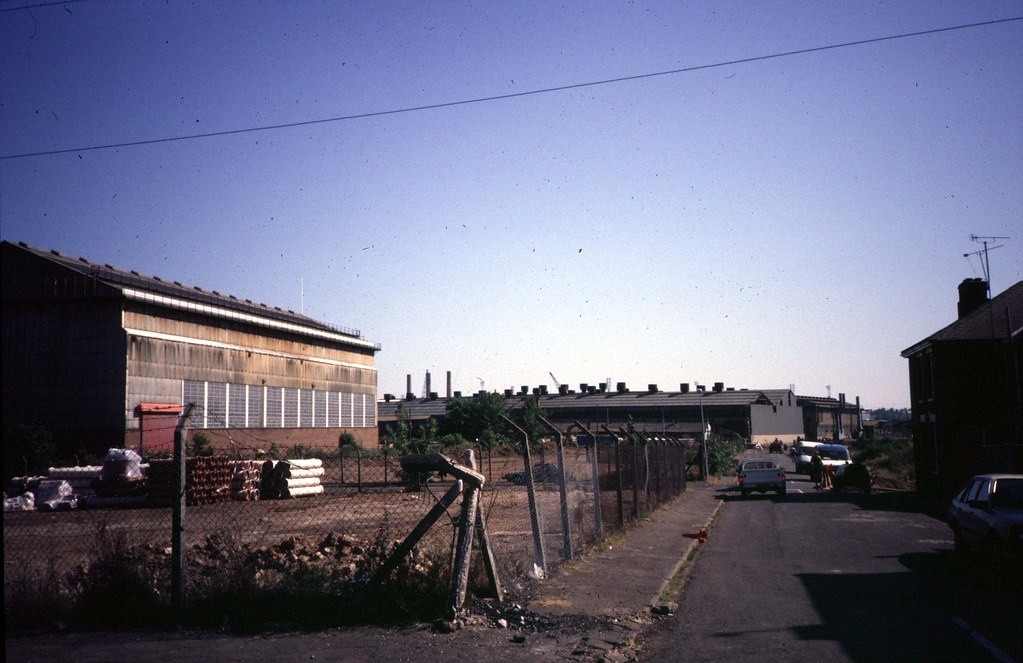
[769,440,784,453]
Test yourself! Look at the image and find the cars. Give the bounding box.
[812,443,850,481]
[949,474,1022,559]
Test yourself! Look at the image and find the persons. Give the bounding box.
[822,437,843,445]
[810,450,823,487]
[775,438,778,442]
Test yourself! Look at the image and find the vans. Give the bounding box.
[790,440,823,472]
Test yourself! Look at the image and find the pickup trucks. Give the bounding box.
[735,460,786,498]
